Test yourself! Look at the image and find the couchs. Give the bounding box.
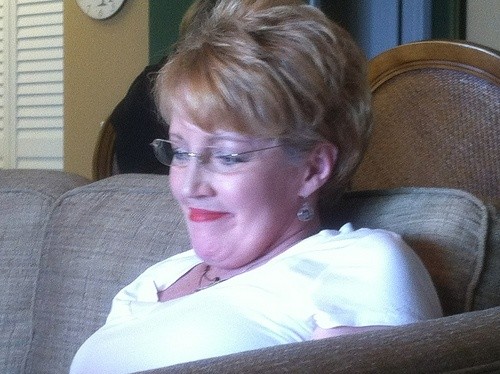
[0,170,500,374]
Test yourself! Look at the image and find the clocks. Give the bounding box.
[76,0,126,21]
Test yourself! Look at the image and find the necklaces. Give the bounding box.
[194,263,225,290]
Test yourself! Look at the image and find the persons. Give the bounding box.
[68,0,441,374]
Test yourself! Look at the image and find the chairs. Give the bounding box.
[92,57,172,180]
[347,39,500,213]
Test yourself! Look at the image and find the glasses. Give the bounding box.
[149,138,316,170]
